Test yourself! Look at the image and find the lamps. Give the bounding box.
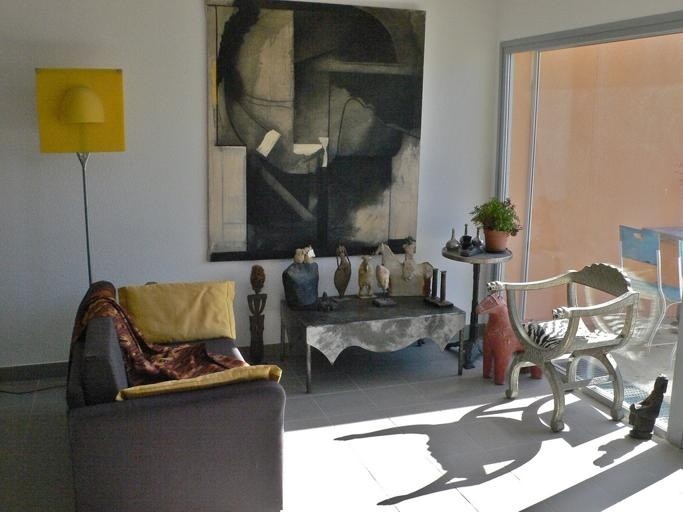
[36,68,126,288]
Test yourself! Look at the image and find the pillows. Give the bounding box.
[82,281,129,405]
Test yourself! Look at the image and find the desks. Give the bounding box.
[442,246,513,369]
[281,293,466,394]
[642,226,682,246]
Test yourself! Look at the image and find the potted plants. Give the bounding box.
[471,197,521,252]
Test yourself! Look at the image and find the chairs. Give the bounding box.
[486,264,639,433]
[618,224,683,352]
[66,281,286,512]
[677,240,683,301]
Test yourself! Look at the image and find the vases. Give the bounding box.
[446,224,484,250]
